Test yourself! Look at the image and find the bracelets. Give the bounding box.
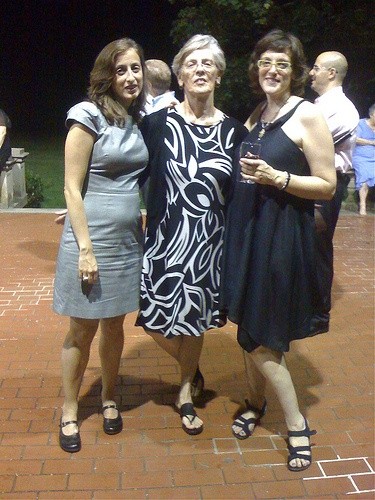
[281,173,293,193]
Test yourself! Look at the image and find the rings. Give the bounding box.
[83,277,89,281]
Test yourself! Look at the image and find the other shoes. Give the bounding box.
[359,202,367,216]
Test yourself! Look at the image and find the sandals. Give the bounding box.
[232,397,267,440]
[288,415,317,471]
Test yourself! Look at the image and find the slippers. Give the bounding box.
[174,402,204,435]
[191,368,205,400]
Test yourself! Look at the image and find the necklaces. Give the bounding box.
[256,94,292,143]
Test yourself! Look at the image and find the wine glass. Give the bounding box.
[239,142,261,184]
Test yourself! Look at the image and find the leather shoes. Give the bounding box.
[101,401,123,434]
[58,415,81,452]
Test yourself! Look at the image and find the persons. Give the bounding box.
[305,50,360,337]
[0,109,14,175]
[234,31,337,471]
[52,37,147,459]
[136,31,231,439]
[352,104,375,215]
[138,57,182,121]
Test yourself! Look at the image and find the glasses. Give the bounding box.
[257,60,293,71]
[313,63,338,73]
